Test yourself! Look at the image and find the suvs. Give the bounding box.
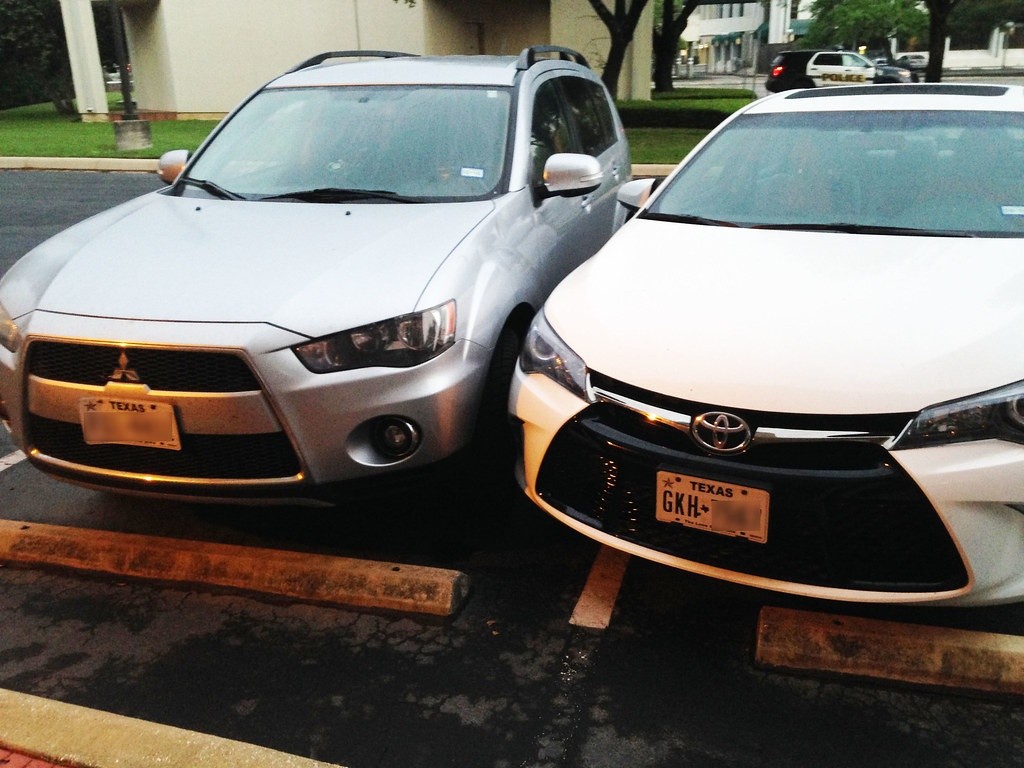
[766,50,913,95]
[0,51,632,528]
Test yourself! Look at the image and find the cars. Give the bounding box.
[896,54,929,69]
[507,83,1023,610]
